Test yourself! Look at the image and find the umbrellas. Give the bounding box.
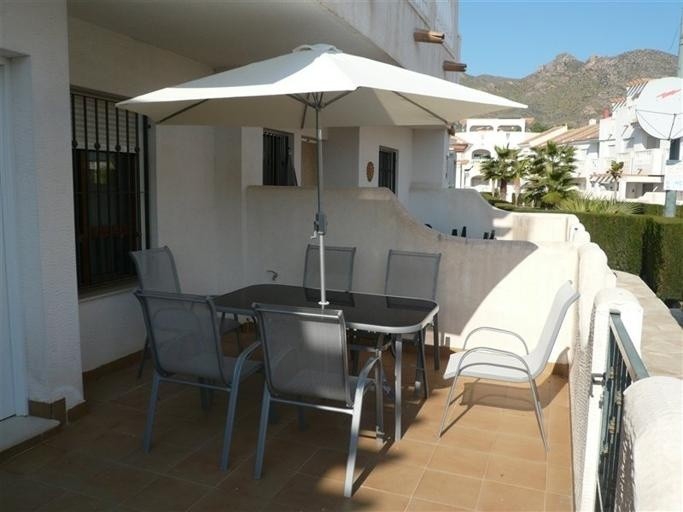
[113,42,528,306]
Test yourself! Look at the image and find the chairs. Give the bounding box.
[130,244,243,379]
[248,302,385,499]
[132,287,267,471]
[302,244,356,292]
[357,248,441,400]
[439,281,581,453]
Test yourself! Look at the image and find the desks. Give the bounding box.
[210,284,438,440]
